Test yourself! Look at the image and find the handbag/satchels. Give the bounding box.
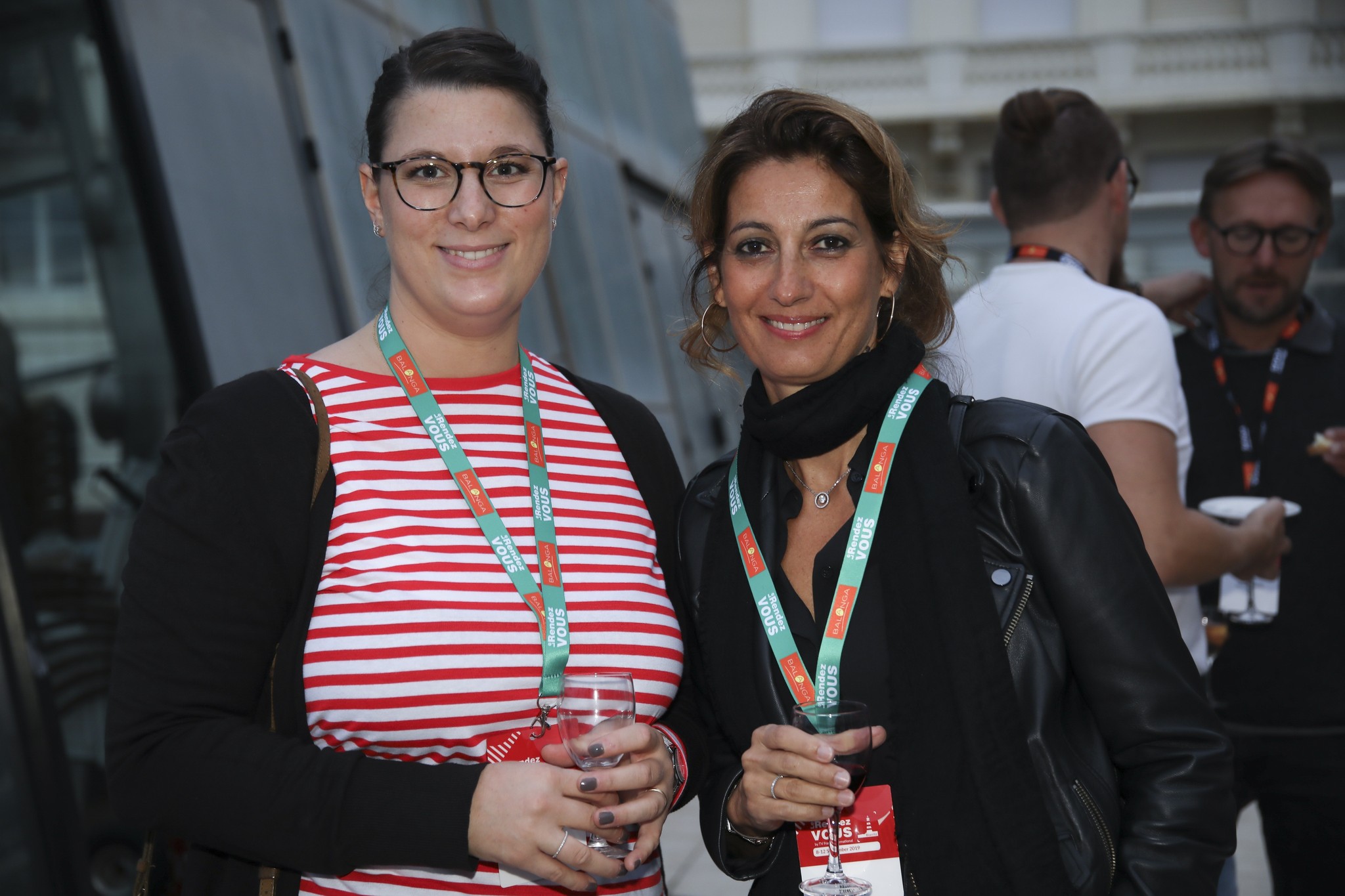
[132,837,302,896]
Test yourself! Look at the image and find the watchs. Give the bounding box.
[659,729,686,801]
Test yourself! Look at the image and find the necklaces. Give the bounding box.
[783,456,853,509]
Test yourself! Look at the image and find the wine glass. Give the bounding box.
[557,670,637,858]
[791,701,873,896]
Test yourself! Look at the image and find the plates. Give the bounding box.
[1198,497,1301,522]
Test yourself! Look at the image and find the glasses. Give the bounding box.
[1202,219,1324,256]
[371,153,557,212]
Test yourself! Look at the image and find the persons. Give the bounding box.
[665,83,1233,894]
[927,87,1293,722]
[1125,136,1345,896]
[106,28,718,893]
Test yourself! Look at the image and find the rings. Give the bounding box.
[639,788,668,809]
[552,830,568,859]
[770,773,787,799]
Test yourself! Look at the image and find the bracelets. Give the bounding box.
[723,777,777,848]
